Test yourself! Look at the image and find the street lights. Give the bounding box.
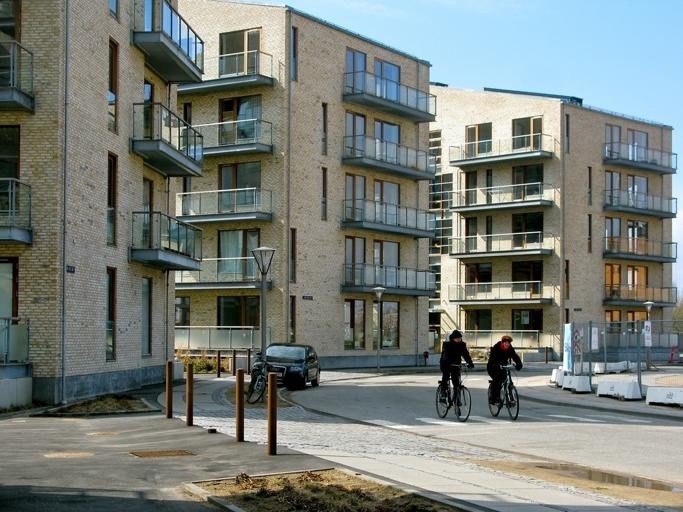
[249,246,277,372]
[641,298,655,372]
[371,285,386,371]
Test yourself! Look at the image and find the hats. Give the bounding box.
[452,330,462,339]
[502,336,513,342]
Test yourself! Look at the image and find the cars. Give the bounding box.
[255,343,320,390]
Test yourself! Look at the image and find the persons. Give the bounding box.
[436,328,474,416]
[485,335,525,410]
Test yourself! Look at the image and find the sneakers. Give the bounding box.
[440,398,447,405]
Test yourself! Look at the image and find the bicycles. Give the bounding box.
[433,361,473,422]
[242,352,275,402]
[486,364,520,422]
